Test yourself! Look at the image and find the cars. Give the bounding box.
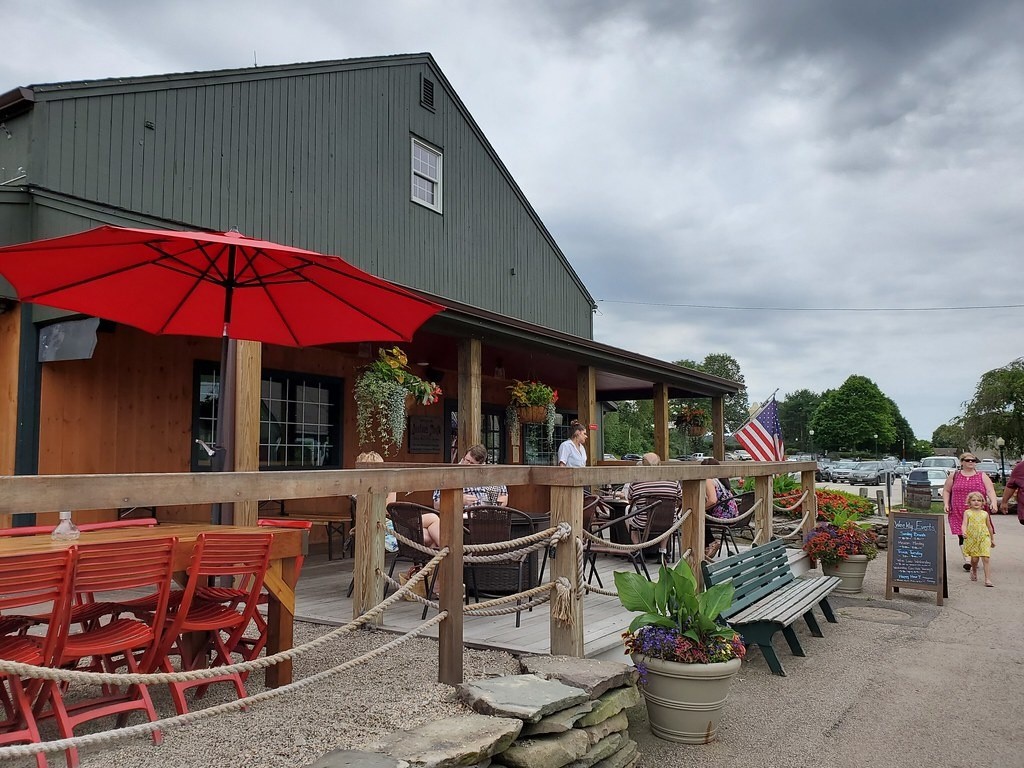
[884,456,921,477]
[901,467,950,502]
[788,470,802,483]
[975,458,1014,481]
[785,452,815,461]
[725,453,736,461]
[604,454,618,460]
[848,461,895,485]
[815,462,832,482]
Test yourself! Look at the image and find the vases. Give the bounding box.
[516,403,548,424]
[821,554,870,593]
[631,651,742,744]
[404,392,418,411]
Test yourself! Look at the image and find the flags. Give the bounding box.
[733,397,785,477]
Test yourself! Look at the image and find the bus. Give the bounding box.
[734,450,752,460]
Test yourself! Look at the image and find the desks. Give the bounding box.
[603,499,671,560]
[0,523,310,690]
[461,511,550,599]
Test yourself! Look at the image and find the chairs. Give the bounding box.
[207,518,312,686]
[627,494,684,571]
[705,491,756,557]
[584,500,663,588]
[0,518,273,768]
[420,505,534,628]
[346,494,430,597]
[383,502,479,604]
[583,490,610,556]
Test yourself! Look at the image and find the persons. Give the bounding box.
[354,451,466,601]
[624,453,682,564]
[558,420,588,468]
[1000,461,1024,526]
[700,458,739,559]
[943,453,998,587]
[433,444,508,510]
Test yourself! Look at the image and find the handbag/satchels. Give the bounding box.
[399,565,433,602]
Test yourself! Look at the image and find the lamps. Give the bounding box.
[424,367,444,383]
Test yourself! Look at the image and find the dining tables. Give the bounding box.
[539,495,603,595]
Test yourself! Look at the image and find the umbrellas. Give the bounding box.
[0,223,447,660]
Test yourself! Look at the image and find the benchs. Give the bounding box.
[288,511,352,561]
[701,537,842,677]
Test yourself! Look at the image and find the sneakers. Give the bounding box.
[705,541,721,558]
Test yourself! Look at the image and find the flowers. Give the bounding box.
[612,553,746,700]
[505,378,558,448]
[350,345,442,457]
[801,508,879,571]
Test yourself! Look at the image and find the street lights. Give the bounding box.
[996,437,1007,485]
[809,430,814,460]
[874,434,878,461]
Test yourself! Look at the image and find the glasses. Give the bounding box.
[963,459,975,462]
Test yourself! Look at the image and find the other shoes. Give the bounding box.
[970,573,977,582]
[628,552,646,564]
[962,563,973,572]
[985,579,994,587]
[657,552,670,563]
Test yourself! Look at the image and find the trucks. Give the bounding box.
[692,453,714,461]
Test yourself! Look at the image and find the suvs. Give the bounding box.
[921,456,961,472]
[676,455,698,461]
[832,462,860,483]
[621,454,643,461]
